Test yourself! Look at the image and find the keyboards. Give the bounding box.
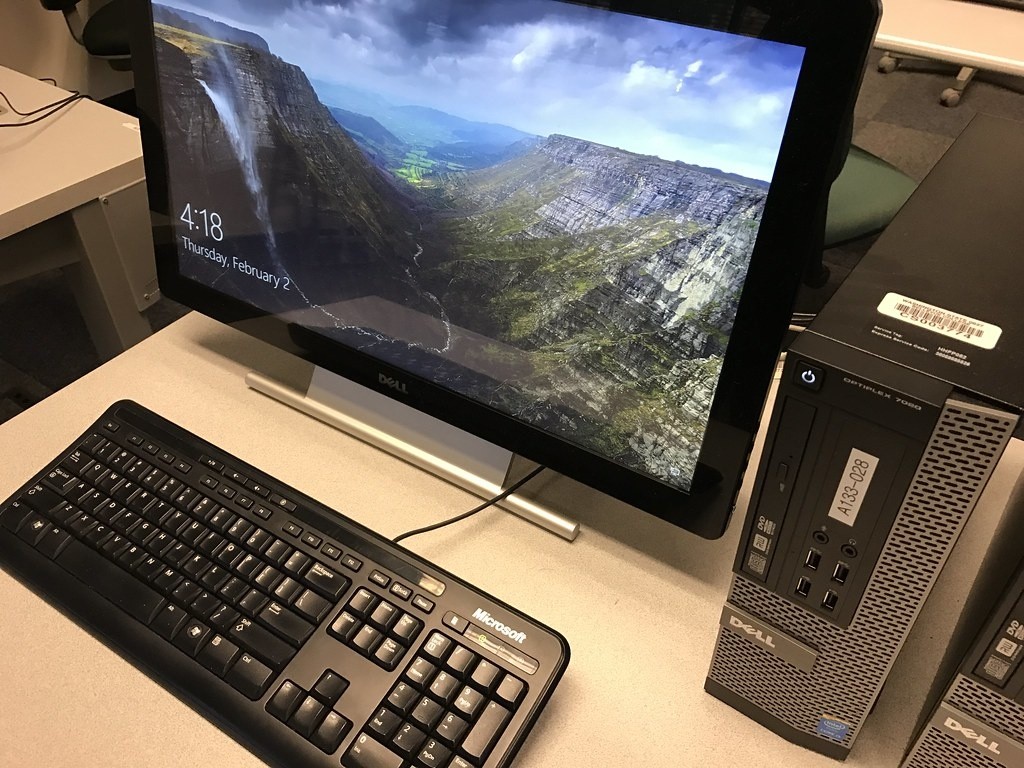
[1,398,570,768]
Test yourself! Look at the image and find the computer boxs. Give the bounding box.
[897,574,1024,768]
[704,111,1023,761]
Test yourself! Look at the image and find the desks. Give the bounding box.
[0,64,163,366]
[0,310,1024,768]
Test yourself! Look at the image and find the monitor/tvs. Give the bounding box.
[130,0,884,542]
